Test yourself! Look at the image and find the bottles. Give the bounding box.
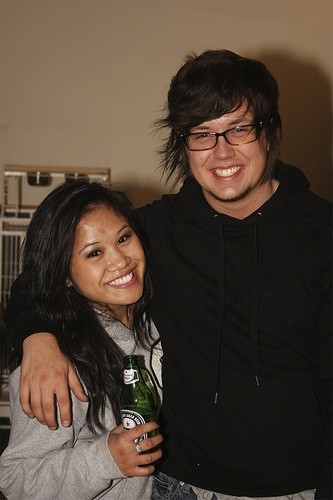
[118,355,160,476]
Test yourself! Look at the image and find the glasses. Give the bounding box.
[171,120,262,152]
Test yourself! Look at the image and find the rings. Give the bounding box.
[135,442,142,454]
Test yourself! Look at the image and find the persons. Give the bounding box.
[0,50,333,500]
[0,178,165,500]
